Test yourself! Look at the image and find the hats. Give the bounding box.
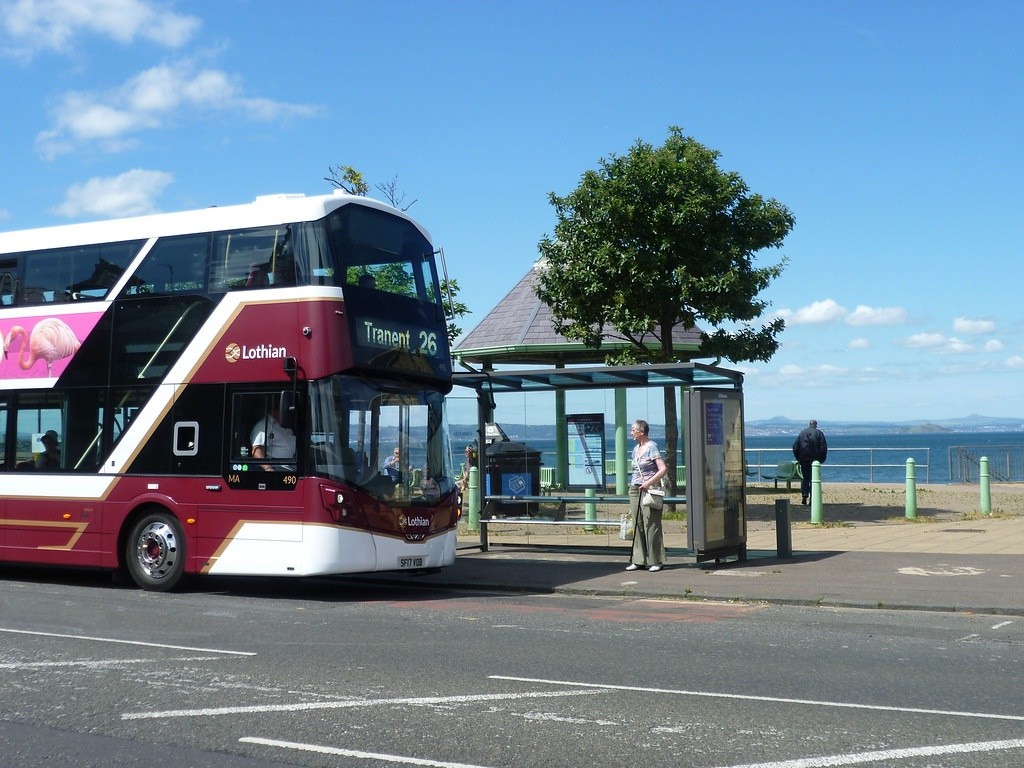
[41,430,59,447]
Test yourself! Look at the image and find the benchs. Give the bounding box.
[539,468,560,506]
[744,459,803,494]
[604,460,635,475]
[477,495,630,526]
[454,462,466,480]
[407,468,418,495]
[676,465,686,491]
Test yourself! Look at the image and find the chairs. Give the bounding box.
[53,290,71,301]
[23,289,46,304]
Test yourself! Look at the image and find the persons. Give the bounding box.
[243,247,377,288]
[251,399,297,461]
[460,445,473,490]
[382,447,405,485]
[792,420,828,505]
[624,419,666,570]
[36,430,61,471]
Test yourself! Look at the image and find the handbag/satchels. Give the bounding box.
[643,488,666,510]
[620,509,635,540]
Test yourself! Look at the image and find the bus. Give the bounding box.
[0,189,459,591]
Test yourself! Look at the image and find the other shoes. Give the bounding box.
[802,497,806,504]
[626,563,646,571]
[649,561,664,572]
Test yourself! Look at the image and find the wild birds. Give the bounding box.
[4,318,81,378]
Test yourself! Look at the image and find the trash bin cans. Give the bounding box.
[485,442,546,520]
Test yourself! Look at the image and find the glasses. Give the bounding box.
[394,452,399,454]
